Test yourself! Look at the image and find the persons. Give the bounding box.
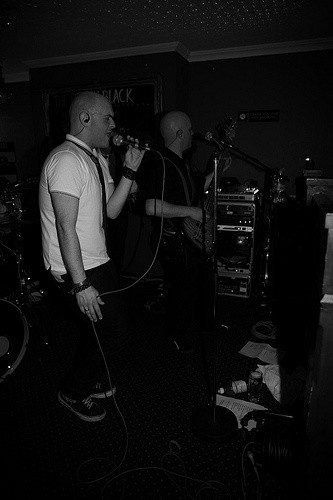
[126,109,231,357]
[40,90,147,422]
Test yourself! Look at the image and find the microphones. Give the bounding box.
[204,132,227,152]
[112,135,154,151]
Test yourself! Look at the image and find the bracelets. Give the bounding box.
[121,166,137,181]
[72,280,91,294]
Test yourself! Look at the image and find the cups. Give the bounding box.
[248,369,263,404]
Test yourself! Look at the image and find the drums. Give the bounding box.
[0,297,29,384]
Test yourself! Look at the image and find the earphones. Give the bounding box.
[85,119,88,122]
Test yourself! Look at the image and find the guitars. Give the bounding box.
[176,117,241,259]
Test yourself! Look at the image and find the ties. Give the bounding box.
[64,140,109,232]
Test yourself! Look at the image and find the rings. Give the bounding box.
[85,309,88,311]
[96,295,100,298]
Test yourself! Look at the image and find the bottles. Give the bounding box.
[217,378,249,395]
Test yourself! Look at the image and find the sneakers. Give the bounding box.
[58,388,105,422]
[82,375,116,399]
